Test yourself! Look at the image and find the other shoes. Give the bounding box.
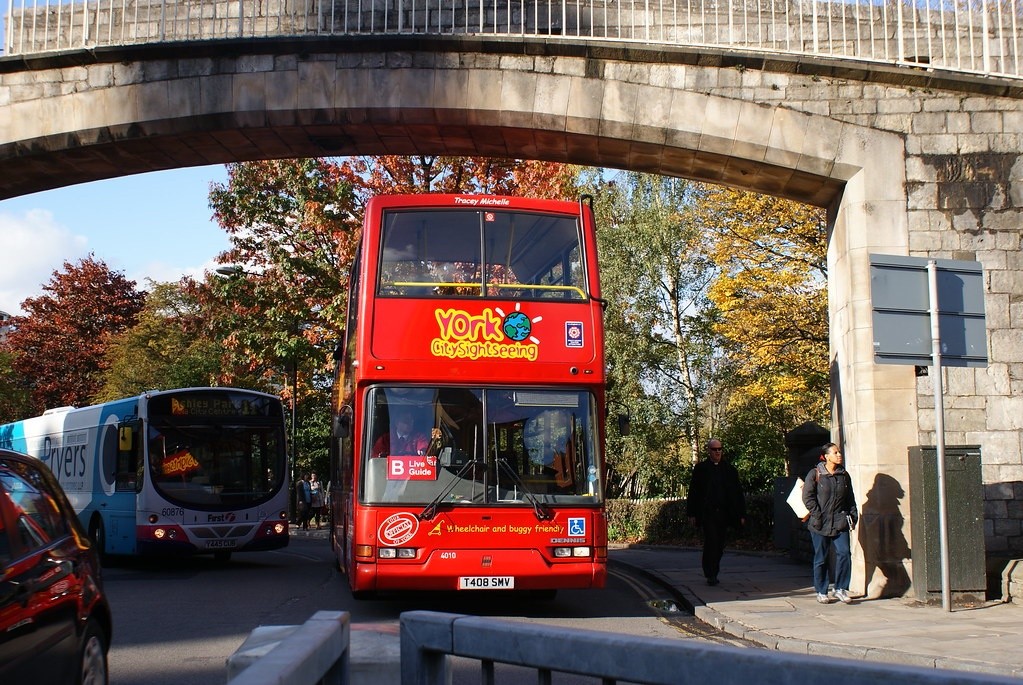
[707,578,720,586]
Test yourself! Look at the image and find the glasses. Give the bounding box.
[709,447,723,451]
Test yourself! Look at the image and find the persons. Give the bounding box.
[801,443,859,605]
[829,473,911,604]
[291,475,311,531]
[688,439,746,586]
[307,473,325,531]
[370,411,430,458]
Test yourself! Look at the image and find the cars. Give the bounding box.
[1,446,115,685]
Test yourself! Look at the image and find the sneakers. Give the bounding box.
[817,592,830,603]
[832,588,853,603]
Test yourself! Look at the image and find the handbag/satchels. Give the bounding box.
[785,468,819,523]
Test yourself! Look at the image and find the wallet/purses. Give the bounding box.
[844,515,855,531]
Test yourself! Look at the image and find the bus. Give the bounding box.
[0,383,307,565]
[318,188,629,607]
